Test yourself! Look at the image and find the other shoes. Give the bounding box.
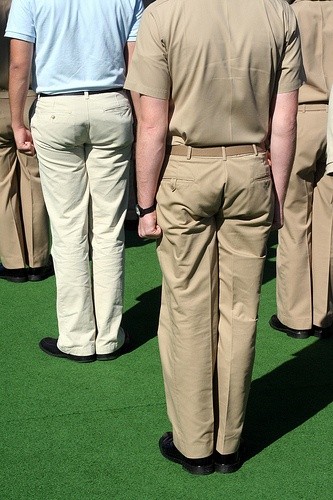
[29,259,54,281]
[0,262,27,282]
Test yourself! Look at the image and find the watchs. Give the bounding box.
[135,202,156,218]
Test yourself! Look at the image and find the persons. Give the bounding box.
[0,0,53,282]
[4,1,149,362]
[268,1,332,340]
[125,0,304,475]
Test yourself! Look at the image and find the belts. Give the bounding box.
[40,87,123,97]
[297,104,328,110]
[0,90,37,99]
[169,144,267,158]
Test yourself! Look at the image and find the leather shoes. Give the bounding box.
[39,337,95,362]
[311,325,333,337]
[270,315,309,339]
[159,432,215,475]
[217,436,246,472]
[96,329,130,360]
